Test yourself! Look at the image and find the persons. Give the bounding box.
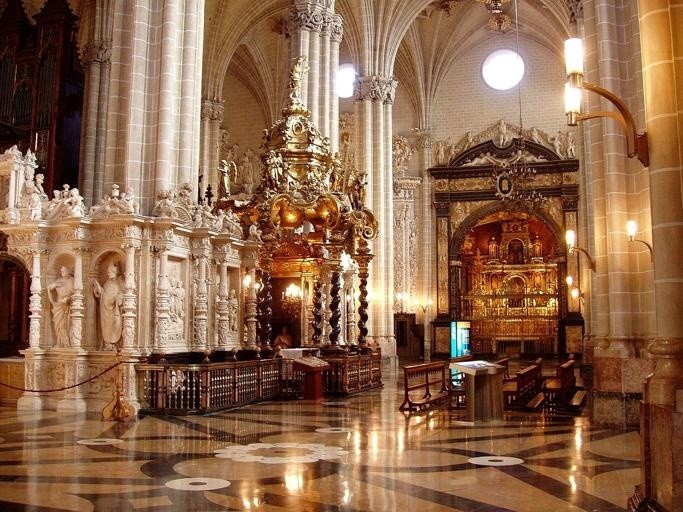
[529,126,544,145]
[547,133,563,154]
[91,260,126,351]
[16,129,265,243]
[564,132,576,159]
[168,277,185,323]
[227,288,239,333]
[44,263,76,348]
[433,139,446,165]
[446,143,458,167]
[458,131,474,153]
[492,118,509,148]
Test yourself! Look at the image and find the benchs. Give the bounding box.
[447,353,589,415]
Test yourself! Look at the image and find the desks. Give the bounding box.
[277,346,322,381]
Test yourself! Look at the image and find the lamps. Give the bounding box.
[416,298,433,314]
[620,216,654,265]
[555,32,650,171]
[563,274,586,307]
[560,227,597,273]
[276,281,302,320]
